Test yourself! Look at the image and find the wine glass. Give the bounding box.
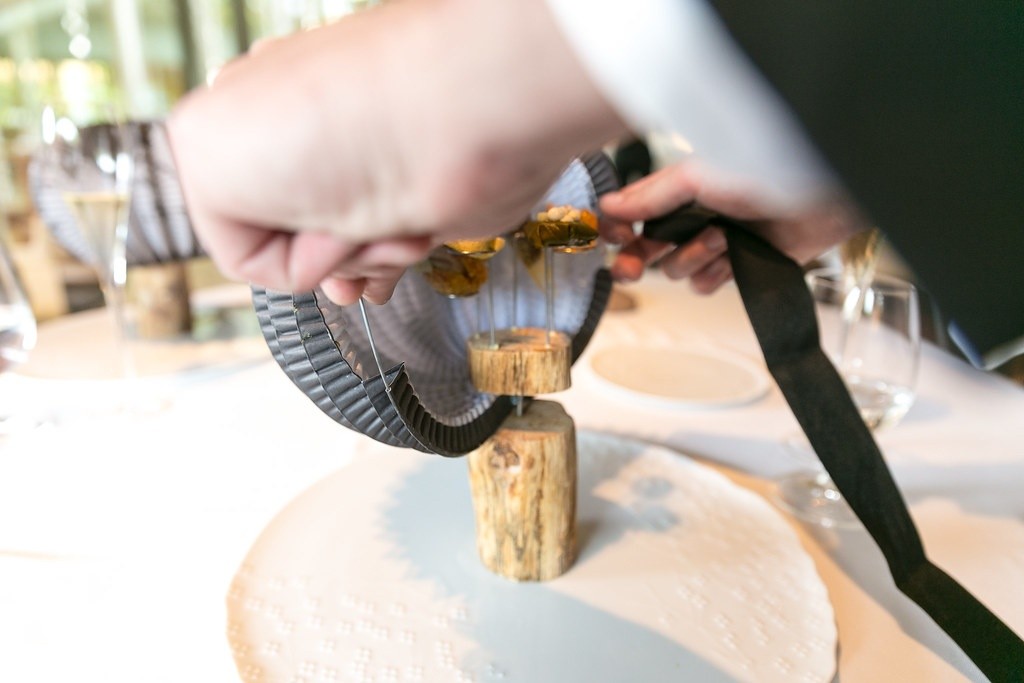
[775,268,924,533]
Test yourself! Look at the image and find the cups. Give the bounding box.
[0,94,201,403]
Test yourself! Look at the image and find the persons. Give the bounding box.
[164,0,1024,391]
